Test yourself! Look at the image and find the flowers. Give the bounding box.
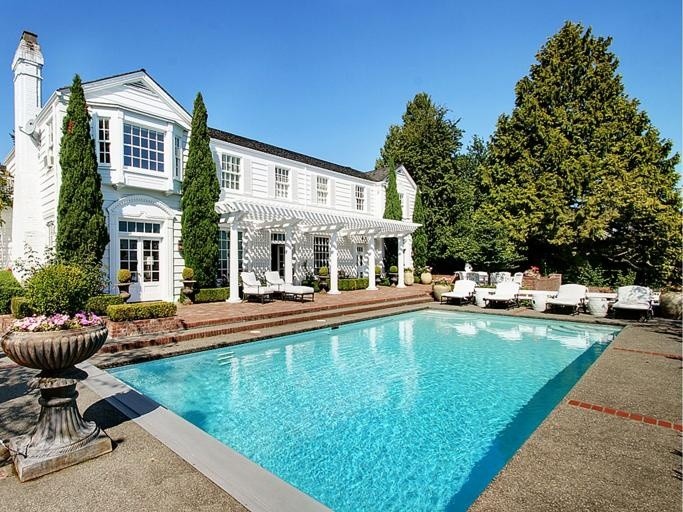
[5,309,104,335]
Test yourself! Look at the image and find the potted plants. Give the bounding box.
[403,266,432,286]
[113,268,133,304]
[179,266,198,306]
[315,265,329,295]
[432,278,451,302]
[375,265,397,289]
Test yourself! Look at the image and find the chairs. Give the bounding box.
[239,269,294,305]
[544,282,589,316]
[439,279,520,309]
[611,284,654,320]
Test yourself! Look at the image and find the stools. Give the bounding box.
[283,285,314,303]
